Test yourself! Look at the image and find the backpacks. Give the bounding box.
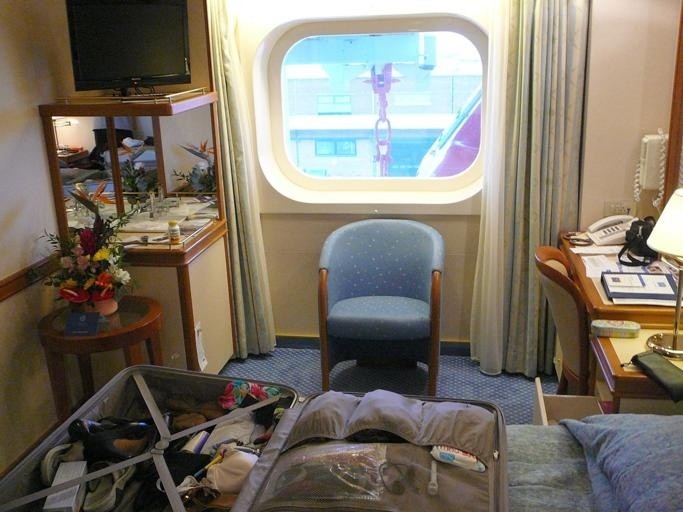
[618,217,656,266]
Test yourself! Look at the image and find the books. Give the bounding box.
[600,271,682,303]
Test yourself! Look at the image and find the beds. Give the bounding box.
[506,414,682,512]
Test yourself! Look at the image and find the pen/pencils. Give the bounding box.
[194,455,222,477]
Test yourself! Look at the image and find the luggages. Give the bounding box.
[0,363,508,512]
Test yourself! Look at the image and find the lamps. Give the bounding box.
[645,188,682,361]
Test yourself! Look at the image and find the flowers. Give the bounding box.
[24,182,151,310]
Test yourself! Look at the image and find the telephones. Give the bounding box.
[587,215,643,245]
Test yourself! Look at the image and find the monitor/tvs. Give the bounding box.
[65,0,192,92]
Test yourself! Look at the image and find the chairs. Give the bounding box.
[533,245,589,395]
[317,218,445,395]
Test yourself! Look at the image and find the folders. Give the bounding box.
[601,272,678,300]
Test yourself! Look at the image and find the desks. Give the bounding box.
[39,296,163,424]
[558,230,683,413]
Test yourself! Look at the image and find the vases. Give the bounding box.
[80,298,119,315]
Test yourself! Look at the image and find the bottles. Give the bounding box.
[168,221,181,246]
